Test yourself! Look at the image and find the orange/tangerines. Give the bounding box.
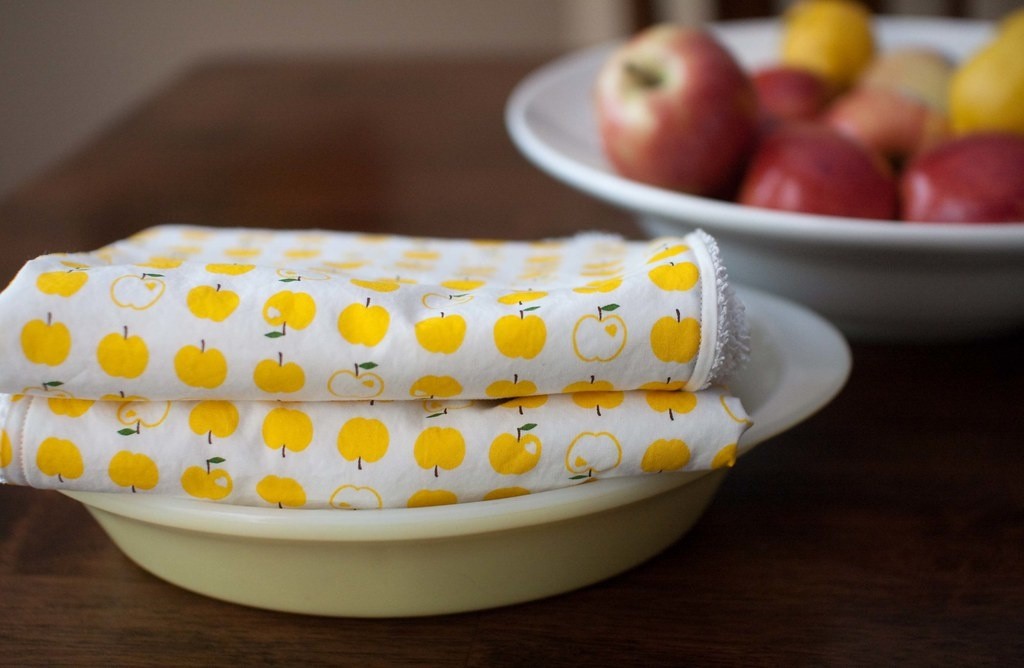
[938,8,1024,136]
[784,0,874,83]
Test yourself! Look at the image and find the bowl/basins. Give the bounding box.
[57,282,851,618]
[505,18,1024,339]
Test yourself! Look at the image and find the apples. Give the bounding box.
[596,21,1024,223]
